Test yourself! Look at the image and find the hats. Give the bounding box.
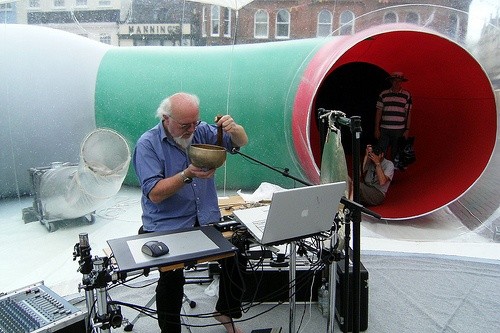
[390,72,408,82]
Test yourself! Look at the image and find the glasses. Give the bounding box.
[392,78,400,83]
[167,115,201,129]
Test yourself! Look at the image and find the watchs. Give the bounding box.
[181,171,193,184]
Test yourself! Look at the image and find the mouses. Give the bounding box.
[141,241,169,257]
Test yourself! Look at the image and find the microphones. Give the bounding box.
[318,108,351,125]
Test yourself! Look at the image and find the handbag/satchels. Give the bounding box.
[396,135,416,169]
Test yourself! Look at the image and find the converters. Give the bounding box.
[214,218,238,231]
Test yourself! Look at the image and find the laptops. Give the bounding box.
[233,181,347,245]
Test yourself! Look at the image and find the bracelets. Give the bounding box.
[405,128,410,130]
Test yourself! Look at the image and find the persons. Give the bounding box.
[375,71,412,170]
[353,138,395,204]
[133,92,249,333]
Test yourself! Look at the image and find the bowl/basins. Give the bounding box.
[188,143,228,169]
